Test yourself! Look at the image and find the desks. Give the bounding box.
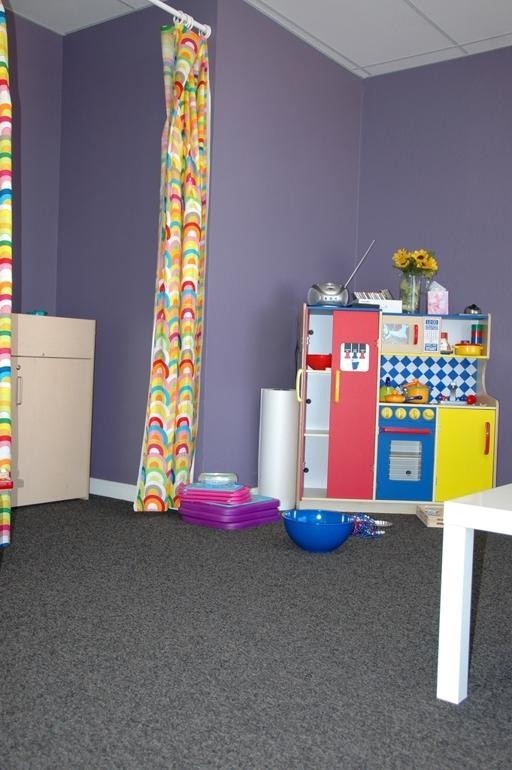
[437,483,512,705]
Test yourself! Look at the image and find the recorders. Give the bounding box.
[306,281,350,306]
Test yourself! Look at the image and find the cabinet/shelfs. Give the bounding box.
[376,404,437,514]
[433,407,497,505]
[10,312,96,509]
[378,312,499,405]
[295,300,380,514]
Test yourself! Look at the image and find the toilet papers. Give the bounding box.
[257,387,299,512]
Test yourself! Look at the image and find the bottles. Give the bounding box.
[380,377,395,402]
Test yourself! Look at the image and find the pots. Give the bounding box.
[403,379,430,403]
[383,389,423,403]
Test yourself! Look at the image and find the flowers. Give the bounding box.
[390,248,438,281]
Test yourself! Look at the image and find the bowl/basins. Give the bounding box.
[281,509,357,553]
[307,355,332,370]
[455,343,483,356]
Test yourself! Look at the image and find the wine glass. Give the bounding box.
[374,520,393,535]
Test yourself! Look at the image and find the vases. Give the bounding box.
[399,271,423,314]
